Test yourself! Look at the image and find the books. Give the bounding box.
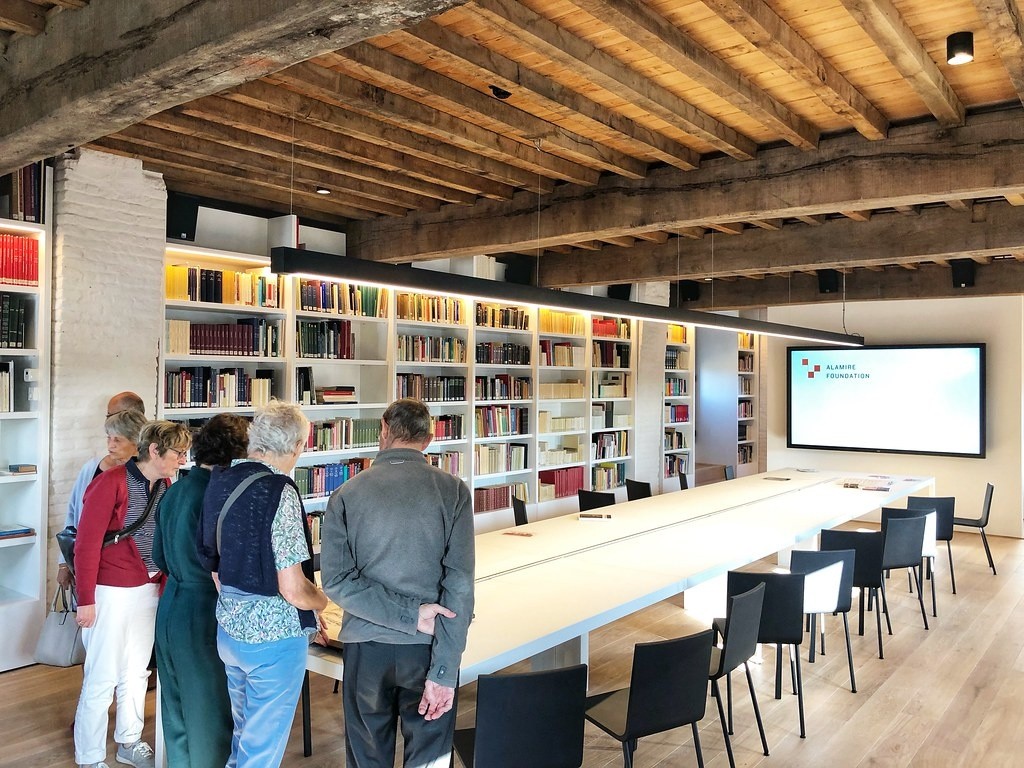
[665,349,689,370]
[665,402,689,478]
[164,266,284,309]
[294,276,633,544]
[0,464,37,476]
[666,323,687,344]
[664,377,687,396]
[0,361,15,413]
[168,416,255,465]
[0,158,44,224]
[738,332,754,350]
[165,366,275,409]
[0,523,36,540]
[0,233,39,287]
[739,375,752,395]
[739,353,754,372]
[0,291,25,348]
[738,399,754,464]
[165,317,287,358]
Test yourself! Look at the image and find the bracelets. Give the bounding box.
[59,566,68,570]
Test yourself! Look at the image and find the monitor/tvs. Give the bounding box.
[786,343,986,459]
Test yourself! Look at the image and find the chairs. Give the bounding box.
[454,483,996,768]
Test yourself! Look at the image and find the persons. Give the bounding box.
[207,399,328,768]
[318,395,476,768]
[88,407,157,692]
[55,390,145,733]
[153,412,251,767]
[71,418,189,768]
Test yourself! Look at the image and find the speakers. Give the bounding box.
[505,255,532,284]
[818,270,838,293]
[679,280,698,302]
[951,259,975,287]
[165,191,199,242]
[606,284,632,300]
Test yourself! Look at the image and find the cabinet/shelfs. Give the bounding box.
[696,328,760,478]
[0,167,54,674]
[157,243,632,536]
[637,320,695,493]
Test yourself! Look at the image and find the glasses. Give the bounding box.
[104,410,126,418]
[169,448,186,459]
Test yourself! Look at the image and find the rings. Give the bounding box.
[79,621,84,625]
[59,584,62,586]
[81,620,85,623]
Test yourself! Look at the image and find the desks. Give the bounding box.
[305,467,841,583]
[302,476,935,768]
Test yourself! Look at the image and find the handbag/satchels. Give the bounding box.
[32,581,86,668]
[55,526,78,575]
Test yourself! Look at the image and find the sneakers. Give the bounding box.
[78,762,109,768]
[116,738,155,768]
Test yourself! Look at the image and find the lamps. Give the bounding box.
[316,186,331,195]
[816,269,838,294]
[948,258,975,289]
[946,31,974,65]
[680,280,700,302]
